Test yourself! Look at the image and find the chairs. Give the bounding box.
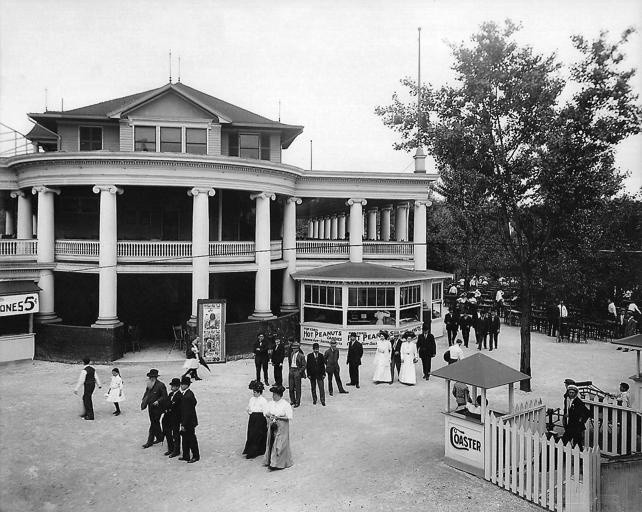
[172,323,184,350]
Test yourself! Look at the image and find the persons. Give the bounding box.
[347,332,363,389]
[618,383,632,408]
[467,396,495,422]
[372,324,436,384]
[452,380,472,406]
[105,368,125,415]
[445,274,520,364]
[563,384,590,465]
[562,378,577,426]
[558,302,567,320]
[627,301,641,316]
[141,337,208,463]
[74,357,102,420]
[243,334,347,471]
[608,300,619,318]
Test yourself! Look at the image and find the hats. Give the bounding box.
[169,376,193,385]
[291,341,301,349]
[272,382,280,387]
[146,369,160,377]
[329,340,338,347]
[564,380,578,393]
[249,380,265,391]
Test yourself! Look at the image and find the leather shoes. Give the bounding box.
[142,439,161,447]
[164,451,197,463]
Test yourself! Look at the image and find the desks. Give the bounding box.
[443,274,642,343]
